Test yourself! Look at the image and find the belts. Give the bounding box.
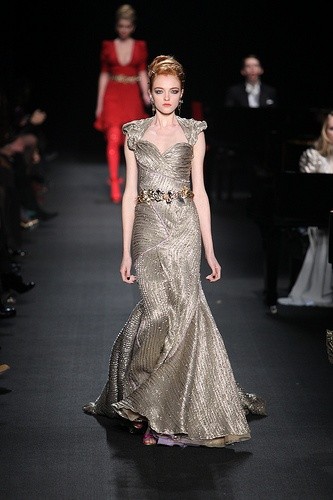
[112,75,140,82]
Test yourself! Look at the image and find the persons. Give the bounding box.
[94,6,151,202]
[226,54,332,317]
[83,56,267,448]
[0,86,59,375]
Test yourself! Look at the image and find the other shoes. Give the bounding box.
[133,421,144,429]
[107,179,122,204]
[0,273,34,318]
[143,433,156,446]
